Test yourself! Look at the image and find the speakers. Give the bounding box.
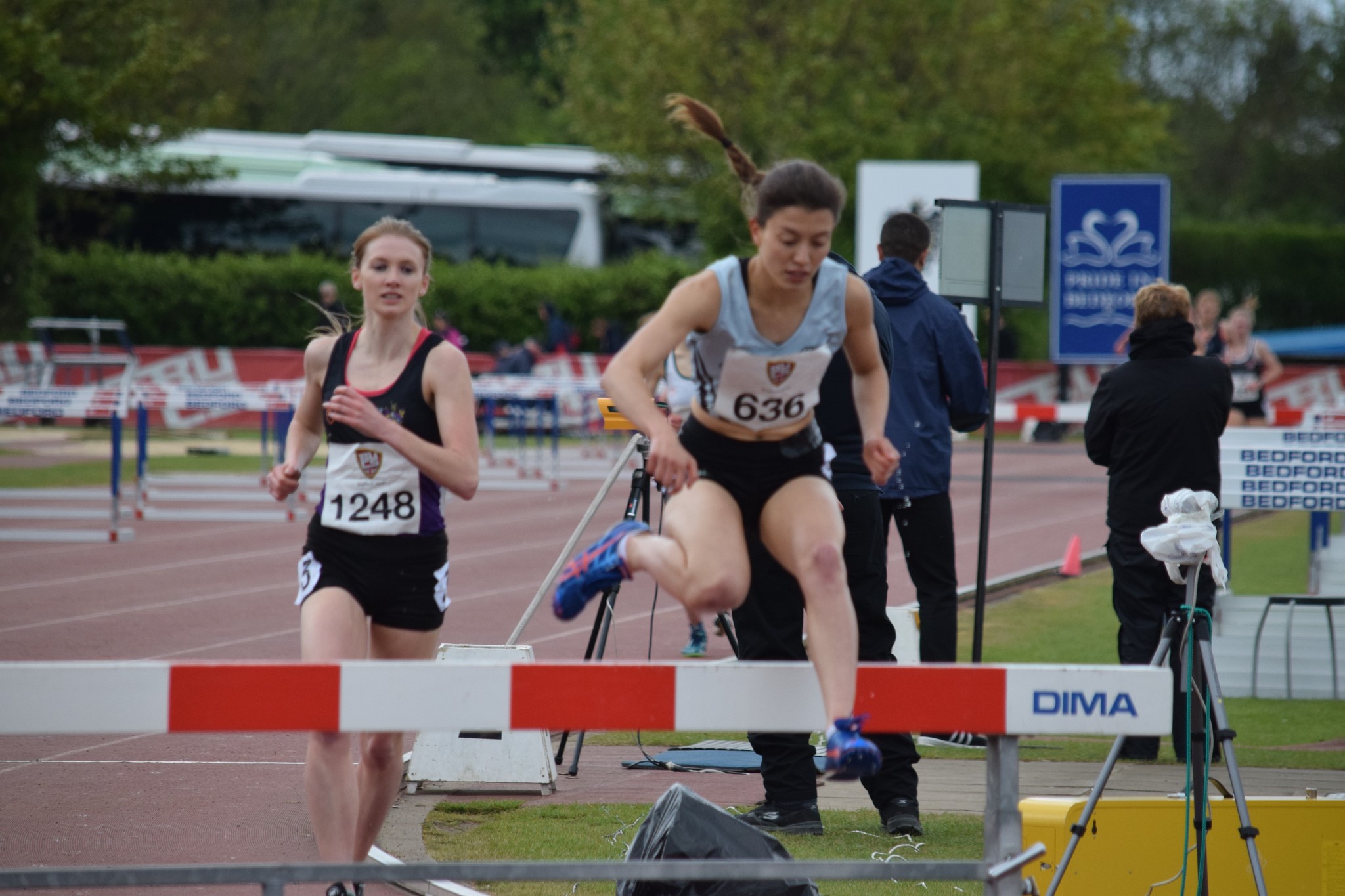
[612,782,821,896]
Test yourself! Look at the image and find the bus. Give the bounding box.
[35,129,692,272]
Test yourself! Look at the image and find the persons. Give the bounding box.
[551,94,900,785]
[735,254,923,837]
[312,283,347,333]
[1191,287,1227,357]
[862,215,989,752]
[1080,280,1233,762]
[432,316,468,351]
[259,217,479,896]
[997,316,1023,366]
[1213,292,1283,420]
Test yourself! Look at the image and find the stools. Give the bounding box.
[1251,593,1345,700]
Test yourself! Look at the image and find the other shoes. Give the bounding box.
[680,620,708,657]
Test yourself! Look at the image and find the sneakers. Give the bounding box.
[881,795,925,836]
[736,799,824,837]
[917,731,988,750]
[823,710,882,782]
[551,520,653,623]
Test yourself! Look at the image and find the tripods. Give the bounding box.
[1045,555,1274,896]
[556,458,739,778]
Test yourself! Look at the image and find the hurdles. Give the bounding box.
[0,651,1175,896]
[1216,422,1344,596]
[0,377,672,546]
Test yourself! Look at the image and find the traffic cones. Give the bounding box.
[1061,535,1083,576]
